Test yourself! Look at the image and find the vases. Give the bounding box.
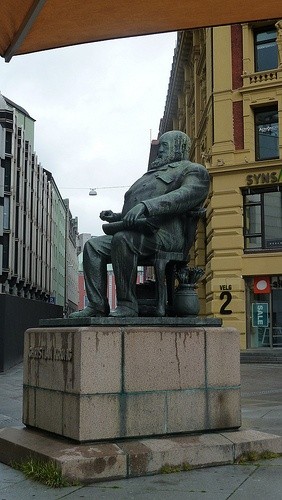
[173,284,200,316]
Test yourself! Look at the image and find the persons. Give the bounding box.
[69,130,210,317]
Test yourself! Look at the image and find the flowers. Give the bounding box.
[176,267,205,283]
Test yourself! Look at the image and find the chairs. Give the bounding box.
[102,206,209,316]
